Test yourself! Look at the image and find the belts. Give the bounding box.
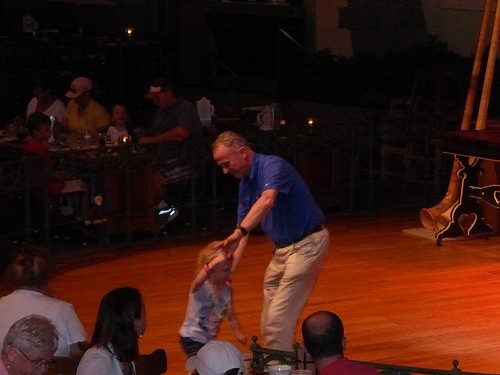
[279,225,323,248]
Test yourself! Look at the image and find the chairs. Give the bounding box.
[0,122,208,240]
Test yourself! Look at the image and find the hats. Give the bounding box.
[144,78,176,100]
[185,339,243,375]
[63,76,94,99]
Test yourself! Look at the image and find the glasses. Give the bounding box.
[17,348,56,368]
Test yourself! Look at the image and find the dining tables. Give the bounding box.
[0,126,165,245]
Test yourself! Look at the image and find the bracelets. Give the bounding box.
[236,225,249,236]
[203,263,214,274]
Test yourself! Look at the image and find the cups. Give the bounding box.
[16,126,28,140]
[7,124,16,139]
[257,105,275,130]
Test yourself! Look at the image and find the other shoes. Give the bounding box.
[75,216,96,231]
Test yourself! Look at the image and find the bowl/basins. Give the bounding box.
[268,364,293,374]
[291,369,313,374]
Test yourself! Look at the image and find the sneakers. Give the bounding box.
[155,204,180,229]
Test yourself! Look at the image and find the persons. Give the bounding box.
[180,241,249,357]
[104,105,134,212]
[54,77,110,134]
[211,131,328,365]
[23,70,67,134]
[0,314,59,375]
[302,311,378,375]
[0,246,90,357]
[25,112,98,232]
[75,287,148,375]
[134,76,202,209]
[186,339,244,375]
